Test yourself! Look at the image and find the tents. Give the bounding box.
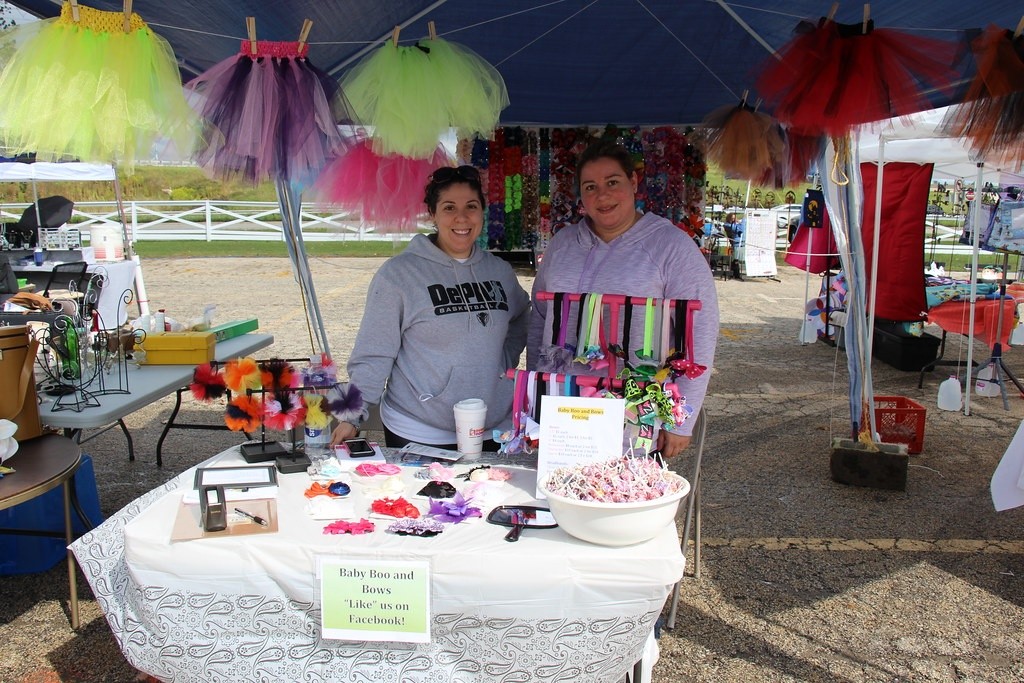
[4,0,1024,440]
[0,149,132,261]
[853,98,1024,414]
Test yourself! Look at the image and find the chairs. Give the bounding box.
[35,261,88,305]
[667,405,706,629]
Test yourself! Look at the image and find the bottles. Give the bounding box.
[303,356,331,461]
[61,316,80,380]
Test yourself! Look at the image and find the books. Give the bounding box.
[334,442,386,467]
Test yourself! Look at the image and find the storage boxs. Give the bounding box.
[852,396,926,453]
[133,332,216,365]
[203,318,259,340]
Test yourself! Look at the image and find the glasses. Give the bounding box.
[427,165,483,184]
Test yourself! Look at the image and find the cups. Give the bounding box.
[34,252,43,266]
[453,398,488,460]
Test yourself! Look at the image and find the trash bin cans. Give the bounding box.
[715,238,731,265]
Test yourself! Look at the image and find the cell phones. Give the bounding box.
[342,437,375,458]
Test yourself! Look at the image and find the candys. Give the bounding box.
[545,436,684,504]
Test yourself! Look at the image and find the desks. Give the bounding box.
[66,440,687,683]
[0,434,82,630]
[4,260,139,329]
[36,334,274,531]
[917,299,1014,390]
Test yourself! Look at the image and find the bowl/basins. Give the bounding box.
[537,470,691,546]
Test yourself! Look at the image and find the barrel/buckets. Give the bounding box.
[937,375,962,412]
[976,364,1000,397]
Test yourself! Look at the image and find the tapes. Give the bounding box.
[206,490,221,506]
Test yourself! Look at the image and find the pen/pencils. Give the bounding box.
[235,508,268,526]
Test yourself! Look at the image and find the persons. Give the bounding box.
[724,213,745,247]
[527,143,720,470]
[329,165,531,451]
